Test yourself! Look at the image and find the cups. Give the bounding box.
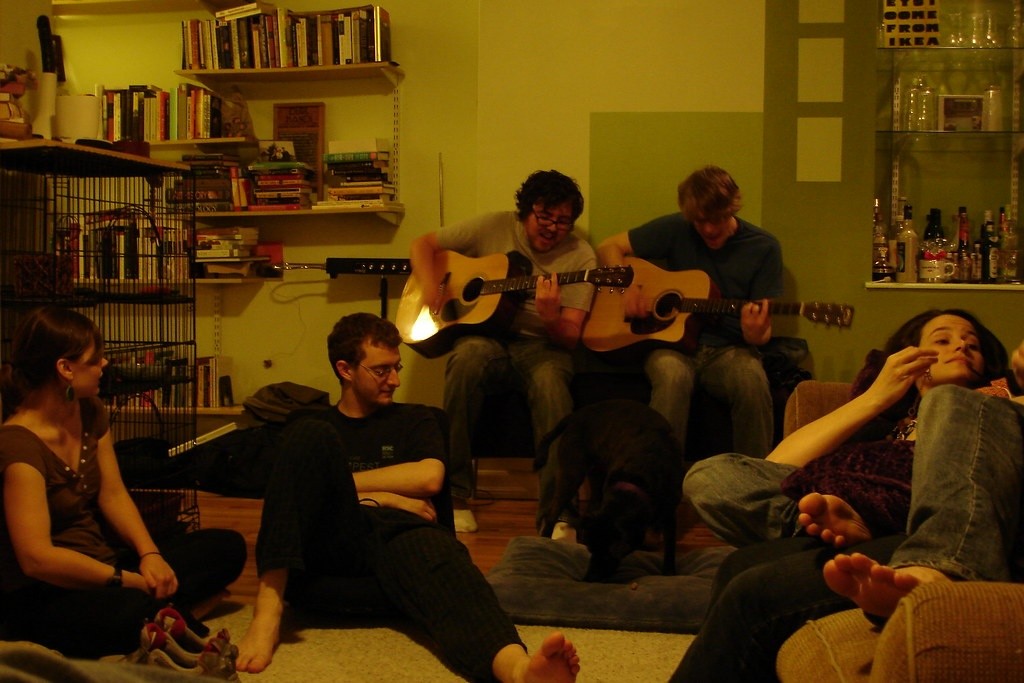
[920,260,954,278]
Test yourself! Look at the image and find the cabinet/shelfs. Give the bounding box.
[73,62,404,417]
[865,43,1024,291]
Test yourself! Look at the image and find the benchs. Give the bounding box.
[467,350,813,462]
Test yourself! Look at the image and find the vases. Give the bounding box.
[55,94,102,145]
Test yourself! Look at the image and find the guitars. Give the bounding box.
[394,249,635,360]
[581,255,857,353]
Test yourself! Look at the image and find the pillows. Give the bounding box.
[486,534,740,640]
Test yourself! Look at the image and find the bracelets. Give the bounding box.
[141,552,161,560]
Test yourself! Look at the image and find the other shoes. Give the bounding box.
[100,607,239,681]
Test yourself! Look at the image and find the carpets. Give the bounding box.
[91,597,697,683]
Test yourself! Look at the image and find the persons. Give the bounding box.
[410,169,598,540]
[236,312,580,683]
[0,306,248,660]
[596,165,787,460]
[670,309,1024,683]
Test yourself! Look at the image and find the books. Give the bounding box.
[312,151,395,209]
[194,227,271,262]
[101,82,223,142]
[182,1,392,70]
[166,154,313,212]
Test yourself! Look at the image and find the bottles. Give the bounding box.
[873,199,918,283]
[906,78,934,130]
[919,207,982,284]
[982,207,1019,282]
[982,85,1005,131]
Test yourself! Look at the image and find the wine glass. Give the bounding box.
[945,0,1024,48]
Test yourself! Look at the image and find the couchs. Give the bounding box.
[773,380,1024,683]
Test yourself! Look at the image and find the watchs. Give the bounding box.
[107,567,123,588]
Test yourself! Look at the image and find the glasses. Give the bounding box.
[532,207,576,231]
[349,357,403,379]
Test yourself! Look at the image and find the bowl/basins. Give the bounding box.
[55,96,98,143]
